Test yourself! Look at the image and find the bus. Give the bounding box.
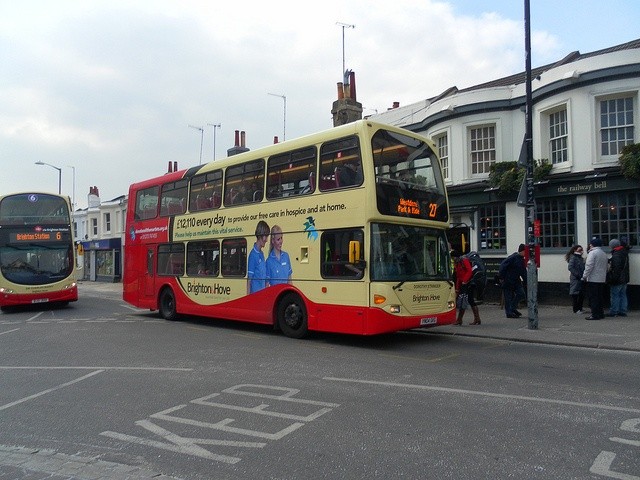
[0,192,78,314]
[121,119,460,337]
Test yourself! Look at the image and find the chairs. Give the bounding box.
[156,204,168,217]
[144,204,157,218]
[253,190,266,202]
[167,199,181,212]
[310,173,326,193]
[333,166,358,188]
[196,193,215,208]
[229,188,244,204]
[212,190,222,207]
[180,197,199,213]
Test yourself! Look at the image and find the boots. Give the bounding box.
[469,306,481,325]
[455,309,464,325]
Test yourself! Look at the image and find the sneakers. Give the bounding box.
[577,310,584,315]
[604,313,616,317]
[506,313,519,318]
[617,313,626,316]
[514,310,521,316]
[585,315,599,319]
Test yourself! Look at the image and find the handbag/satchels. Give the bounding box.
[456,294,468,308]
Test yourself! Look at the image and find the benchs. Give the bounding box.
[453,258,522,309]
[170,251,204,273]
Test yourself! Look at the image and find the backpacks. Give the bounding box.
[455,252,486,289]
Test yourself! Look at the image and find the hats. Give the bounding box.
[589,237,602,246]
[609,239,620,248]
[519,244,525,252]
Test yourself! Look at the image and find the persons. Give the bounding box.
[566,245,586,314]
[247,221,270,296]
[582,236,608,320]
[450,250,481,326]
[265,225,292,288]
[498,243,527,318]
[607,238,630,317]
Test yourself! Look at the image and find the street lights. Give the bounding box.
[335,22,355,80]
[186,125,204,167]
[209,122,221,159]
[268,93,287,142]
[36,161,63,201]
